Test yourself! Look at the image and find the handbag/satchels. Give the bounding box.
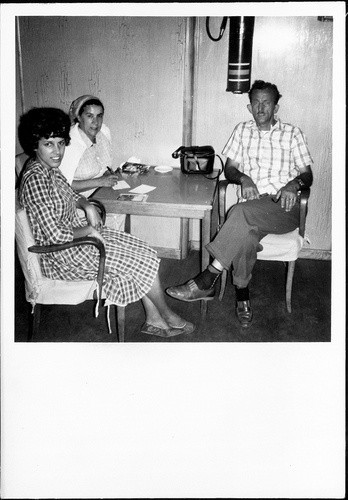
[171,145,224,181]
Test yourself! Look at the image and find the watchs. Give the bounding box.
[295,178,306,188]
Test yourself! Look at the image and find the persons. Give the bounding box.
[59,94,126,231]
[167,80,314,329]
[18,107,196,338]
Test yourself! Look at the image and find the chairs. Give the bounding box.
[15,198,126,342]
[216,180,311,316]
[15,152,32,177]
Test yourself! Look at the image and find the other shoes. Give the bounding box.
[139,320,187,337]
[168,320,196,334]
[166,278,216,302]
[236,298,253,329]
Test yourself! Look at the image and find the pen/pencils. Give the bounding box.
[107,166,116,177]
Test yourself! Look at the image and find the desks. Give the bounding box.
[85,165,226,321]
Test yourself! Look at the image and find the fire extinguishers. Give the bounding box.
[206,16,255,94]
